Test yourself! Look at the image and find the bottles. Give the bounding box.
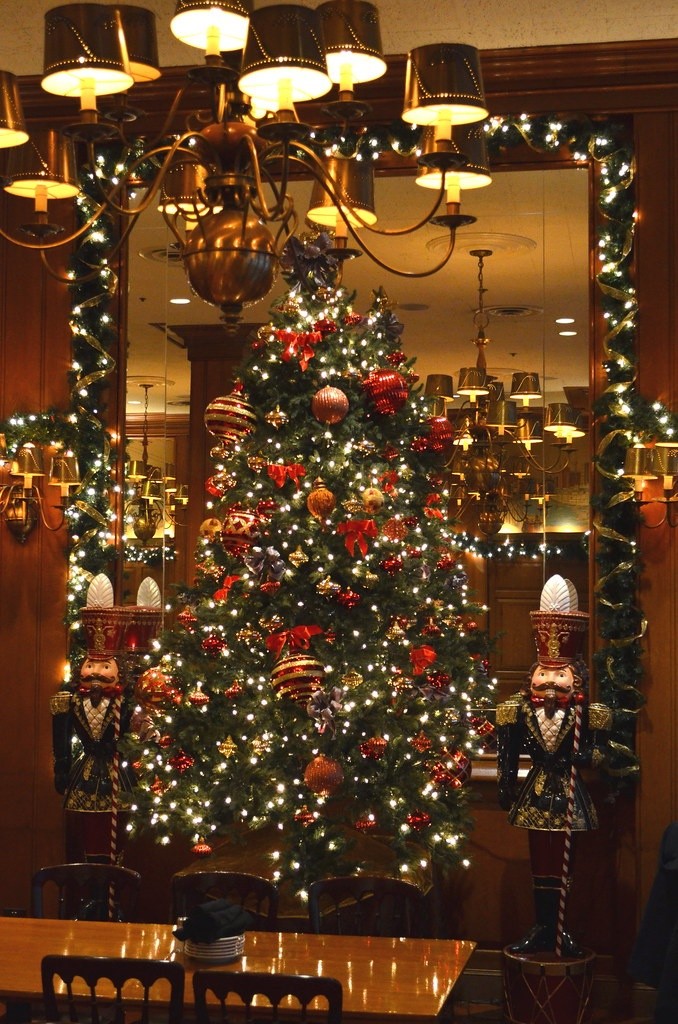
[175,917,187,952]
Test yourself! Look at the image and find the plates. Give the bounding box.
[183,934,245,963]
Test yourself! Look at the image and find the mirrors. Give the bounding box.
[47,115,647,806]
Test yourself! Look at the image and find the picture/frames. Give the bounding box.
[485,424,590,542]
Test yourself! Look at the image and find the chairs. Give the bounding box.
[172,871,280,932]
[192,970,343,1024]
[308,876,422,938]
[30,863,142,924]
[40,954,186,1024]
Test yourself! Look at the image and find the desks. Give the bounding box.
[1,916,478,1024]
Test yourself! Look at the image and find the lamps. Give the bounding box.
[124,384,188,546]
[621,441,678,528]
[0,0,493,337]
[423,247,590,542]
[0,433,82,544]
